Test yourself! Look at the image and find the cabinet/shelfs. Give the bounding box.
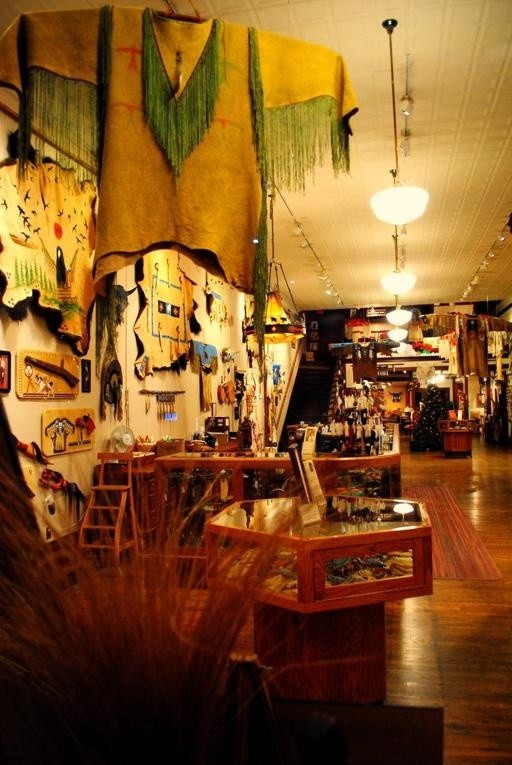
[94,440,240,547]
[154,423,401,586]
[439,419,475,459]
[204,495,434,706]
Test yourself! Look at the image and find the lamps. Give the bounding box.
[388,329,408,342]
[371,185,430,225]
[386,310,412,327]
[380,273,416,295]
[398,92,414,117]
